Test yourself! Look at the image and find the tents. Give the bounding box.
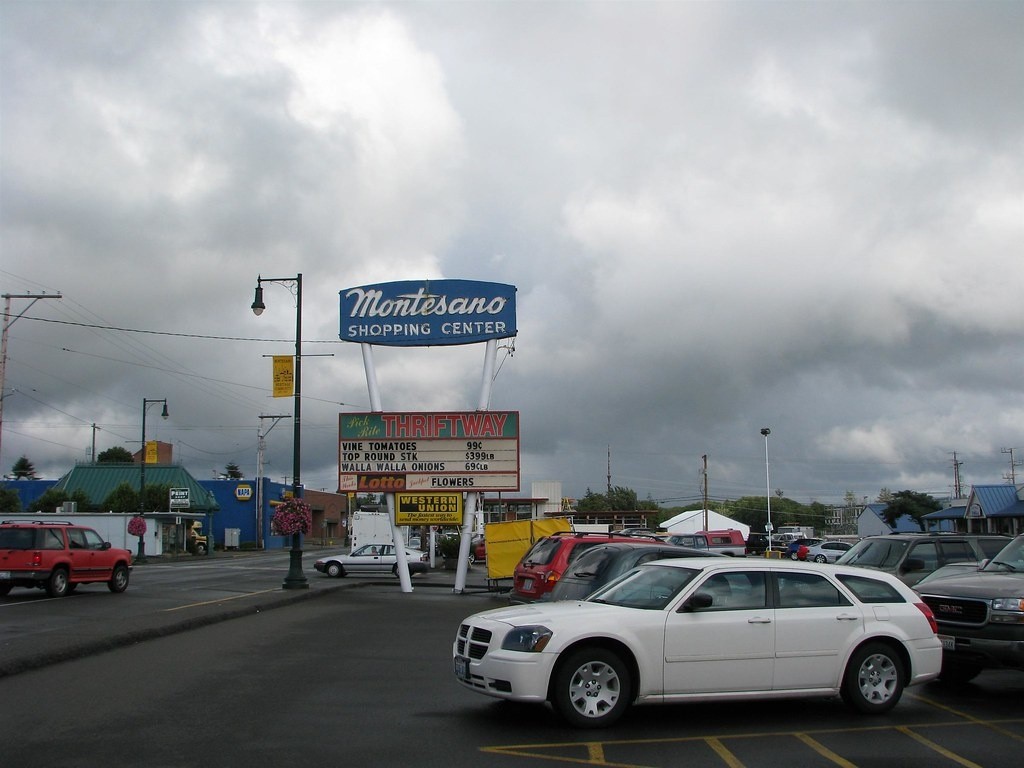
[660,509,750,540]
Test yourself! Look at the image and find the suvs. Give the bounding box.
[745,532,807,556]
[911,530,1024,687]
[0,520,134,600]
[451,556,944,732]
[611,527,667,540]
[835,532,1014,588]
[508,531,666,605]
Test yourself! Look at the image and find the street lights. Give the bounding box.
[134,397,170,563]
[760,427,772,552]
[250,273,310,591]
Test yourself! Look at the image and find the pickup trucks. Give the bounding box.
[664,528,746,558]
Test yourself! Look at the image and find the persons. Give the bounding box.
[786,541,810,561]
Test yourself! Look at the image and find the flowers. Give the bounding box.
[271,495,312,535]
[127,518,147,535]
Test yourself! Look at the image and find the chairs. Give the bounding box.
[711,576,732,606]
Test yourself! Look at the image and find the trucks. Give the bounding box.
[352,511,409,553]
[191,521,208,552]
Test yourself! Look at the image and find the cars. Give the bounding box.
[314,543,430,579]
[548,543,731,603]
[785,537,824,561]
[409,532,487,564]
[918,558,991,585]
[805,541,854,564]
